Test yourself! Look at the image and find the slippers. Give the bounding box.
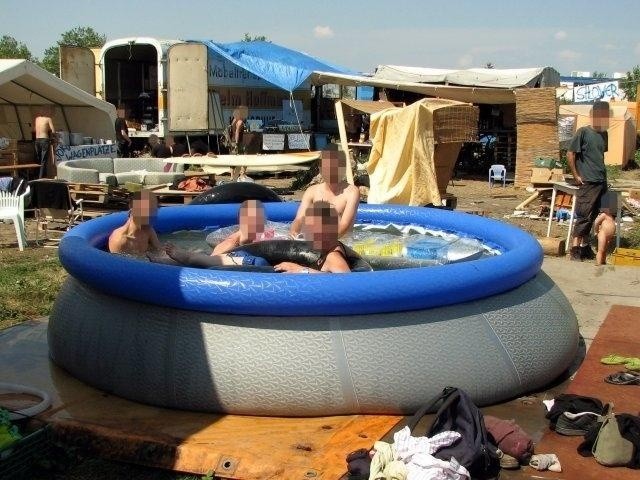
[600,354,639,365]
[604,371,640,386]
[624,361,640,370]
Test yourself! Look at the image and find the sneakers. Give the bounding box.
[580,243,595,259]
[571,245,583,262]
[555,410,600,436]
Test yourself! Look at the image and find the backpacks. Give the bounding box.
[345,385,499,480]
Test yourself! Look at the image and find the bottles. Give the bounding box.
[93,136,99,144]
[244,121,250,132]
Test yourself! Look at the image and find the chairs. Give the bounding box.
[30,181,84,248]
[0,176,31,252]
[489,165,506,189]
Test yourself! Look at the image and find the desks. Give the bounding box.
[547,183,621,251]
[334,142,372,162]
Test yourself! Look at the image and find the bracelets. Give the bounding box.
[303,267,309,273]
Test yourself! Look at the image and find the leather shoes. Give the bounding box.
[500,453,520,469]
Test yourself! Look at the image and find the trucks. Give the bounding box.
[60,37,362,158]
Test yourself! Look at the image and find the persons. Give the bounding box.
[113,103,216,157]
[208,199,266,256]
[593,192,619,266]
[164,200,352,273]
[34,103,55,179]
[565,100,610,262]
[288,147,360,240]
[108,188,161,254]
[228,106,249,180]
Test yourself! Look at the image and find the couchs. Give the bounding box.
[57,157,194,187]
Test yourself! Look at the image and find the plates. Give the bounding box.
[83,135,94,139]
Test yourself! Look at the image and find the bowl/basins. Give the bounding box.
[264,126,277,134]
[277,123,303,133]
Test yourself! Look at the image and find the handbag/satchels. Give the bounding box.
[592,400,635,468]
[220,124,233,149]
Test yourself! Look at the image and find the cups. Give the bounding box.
[106,139,112,145]
[99,138,105,145]
